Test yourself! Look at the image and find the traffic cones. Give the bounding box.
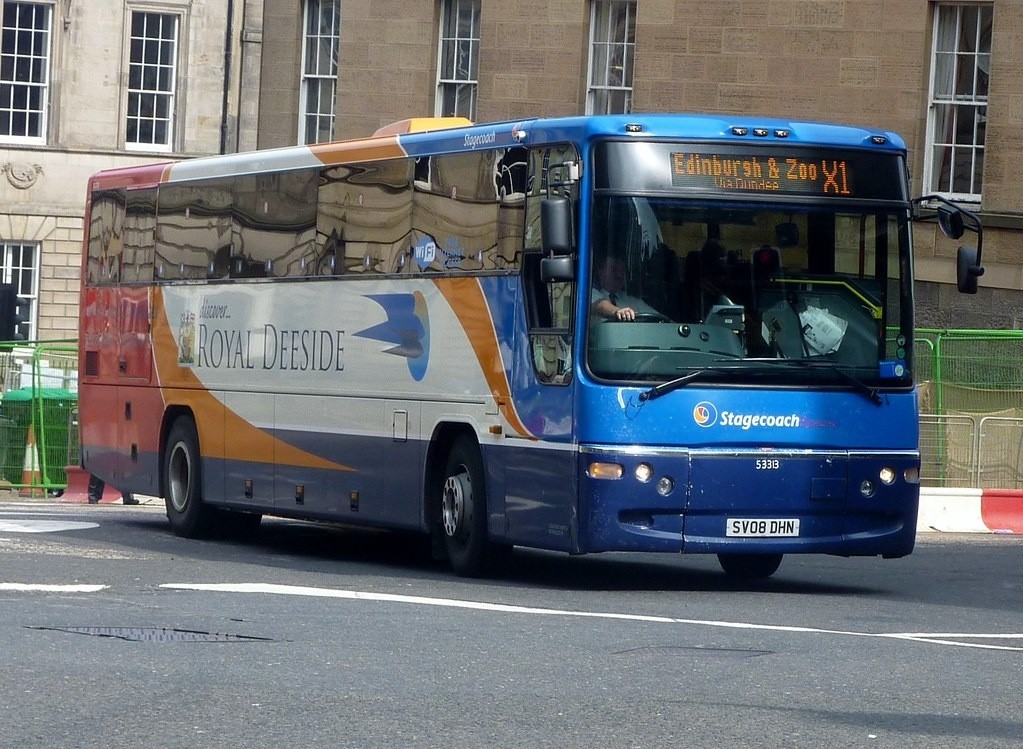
[18,422,46,497]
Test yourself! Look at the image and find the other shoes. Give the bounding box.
[123,499,139,505]
[88,500,98,503]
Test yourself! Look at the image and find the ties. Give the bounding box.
[608,293,618,307]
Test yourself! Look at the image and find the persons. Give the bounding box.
[698,239,728,289]
[590,251,674,323]
[88,474,139,505]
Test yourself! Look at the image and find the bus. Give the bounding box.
[72,114,984,583]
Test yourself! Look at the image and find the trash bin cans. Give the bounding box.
[0,387,79,498]
